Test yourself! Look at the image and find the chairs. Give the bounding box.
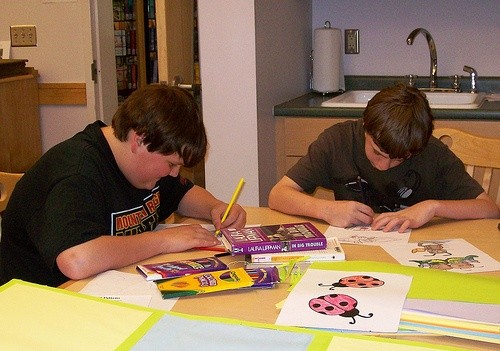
[431,127,500,207]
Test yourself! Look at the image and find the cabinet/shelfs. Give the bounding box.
[274,116,500,206]
[112,0,157,110]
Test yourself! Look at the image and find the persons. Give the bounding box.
[267,84,500,232]
[0,84,247,289]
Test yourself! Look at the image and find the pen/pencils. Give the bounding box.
[215,178,246,236]
[357,175,367,206]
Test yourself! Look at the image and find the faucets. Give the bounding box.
[406,28,437,84]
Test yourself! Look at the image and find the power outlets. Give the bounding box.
[10,25,38,47]
[345,28,360,54]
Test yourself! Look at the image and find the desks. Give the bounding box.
[24,204,500,351]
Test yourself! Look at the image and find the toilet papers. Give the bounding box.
[312,29,345,93]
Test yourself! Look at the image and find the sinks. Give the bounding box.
[322,90,485,109]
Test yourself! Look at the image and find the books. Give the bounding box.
[220,222,327,257]
[245,237,345,265]
